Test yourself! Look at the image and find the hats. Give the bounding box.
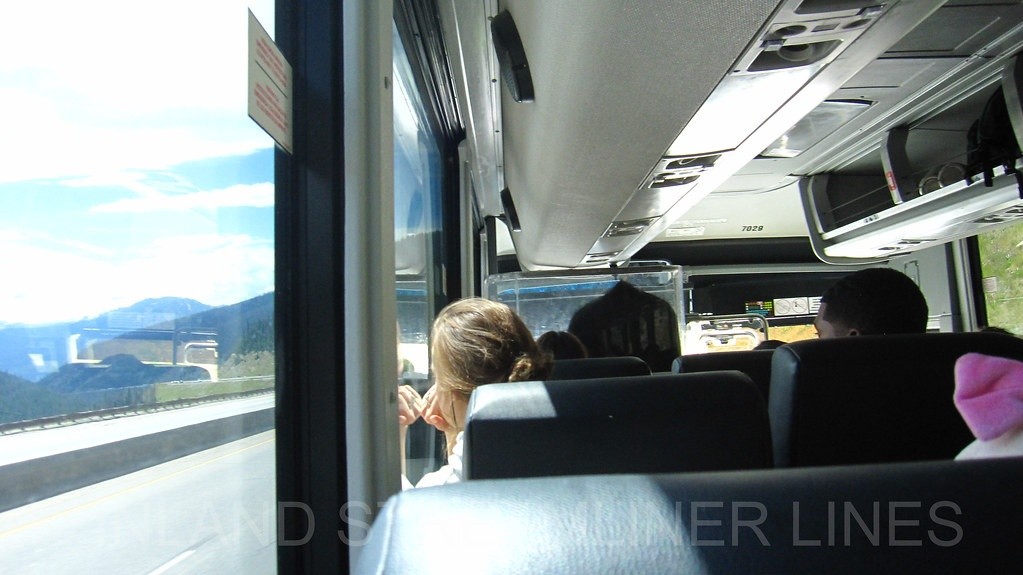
[954,355,1022,459]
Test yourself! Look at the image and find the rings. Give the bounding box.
[425,398,431,406]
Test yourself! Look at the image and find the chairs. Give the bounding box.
[350,328,1023,575]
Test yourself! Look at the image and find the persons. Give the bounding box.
[396,321,421,491]
[414,297,558,489]
[536,330,586,360]
[814,269,929,338]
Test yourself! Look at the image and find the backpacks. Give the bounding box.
[965,86,1021,175]
